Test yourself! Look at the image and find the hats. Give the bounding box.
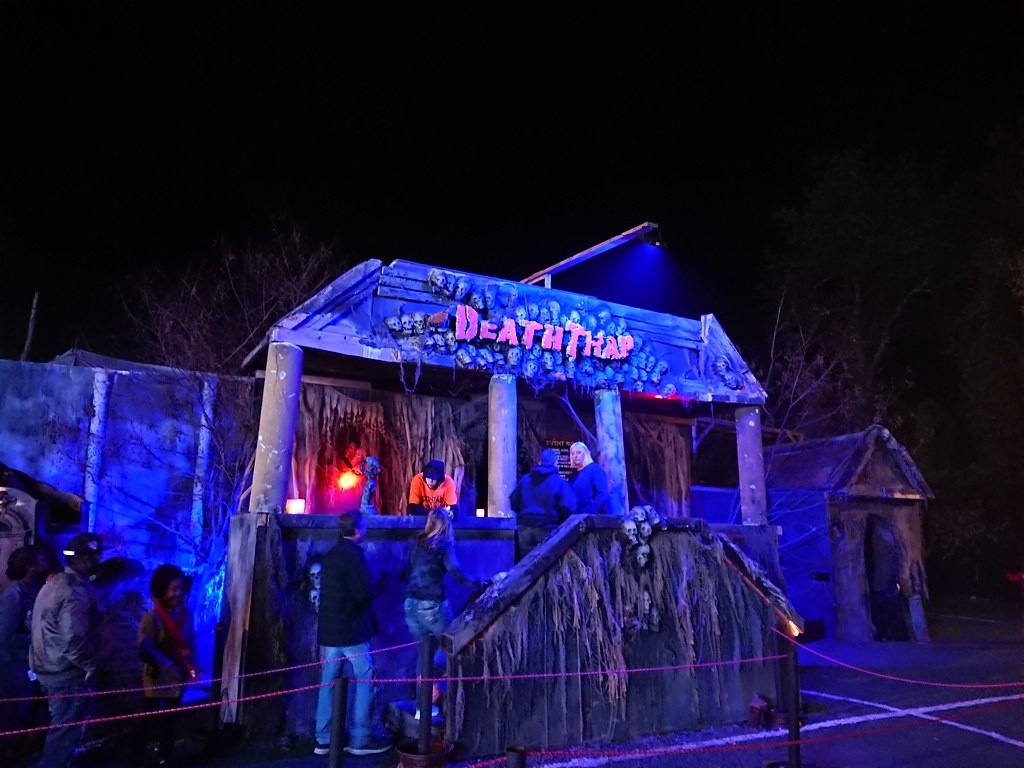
[62,533,102,556]
[423,460,445,480]
[540,449,558,466]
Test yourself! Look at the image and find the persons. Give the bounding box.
[507,447,578,554]
[402,509,507,720]
[133,564,195,764]
[0,534,115,768]
[409,459,458,515]
[314,510,392,755]
[563,441,608,517]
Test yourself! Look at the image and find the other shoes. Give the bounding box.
[348,737,392,755]
[314,744,330,755]
